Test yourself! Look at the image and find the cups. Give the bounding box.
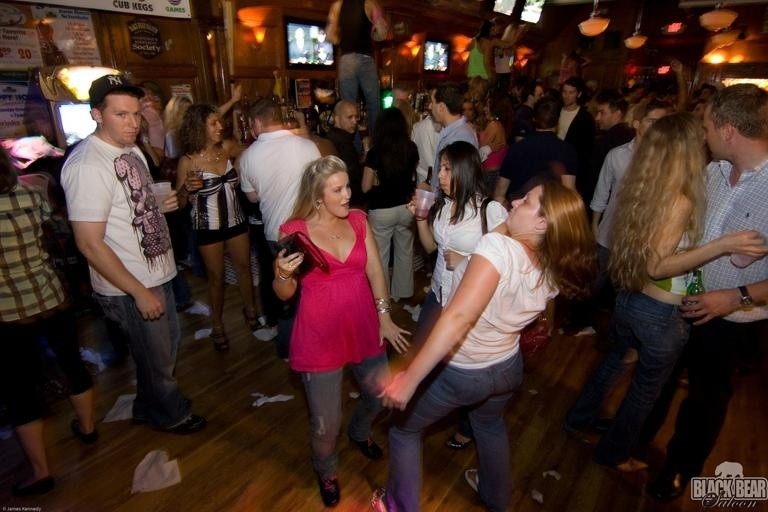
[414,189,435,220]
[730,230,768,270]
[186,170,203,180]
[150,182,171,214]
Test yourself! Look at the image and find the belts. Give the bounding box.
[2,301,71,325]
[641,283,686,308]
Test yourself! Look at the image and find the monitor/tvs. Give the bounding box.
[58,103,97,146]
[520,0,545,24]
[493,0,516,16]
[284,17,337,70]
[424,39,448,71]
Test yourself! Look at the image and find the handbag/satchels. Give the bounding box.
[481,196,497,234]
[521,307,557,375]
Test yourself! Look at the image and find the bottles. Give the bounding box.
[424,167,433,188]
[240,95,297,142]
[686,266,705,318]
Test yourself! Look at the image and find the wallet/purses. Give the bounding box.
[273,232,331,278]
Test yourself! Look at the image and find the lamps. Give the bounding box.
[701,1,736,33]
[579,1,609,37]
[251,27,266,51]
[624,9,646,48]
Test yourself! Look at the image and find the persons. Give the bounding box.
[585,81,598,118]
[409,140,508,448]
[563,91,637,225]
[411,103,444,188]
[60,75,205,434]
[463,96,476,121]
[429,82,480,200]
[274,154,413,506]
[362,107,420,305]
[478,98,509,195]
[63,142,131,366]
[637,85,768,498]
[464,13,591,88]
[0,144,98,498]
[133,115,209,317]
[494,95,577,207]
[561,80,765,472]
[174,104,278,350]
[162,83,244,267]
[556,78,594,142]
[327,1,392,112]
[372,178,598,512]
[564,98,673,336]
[236,99,324,362]
[325,101,356,162]
[512,77,559,138]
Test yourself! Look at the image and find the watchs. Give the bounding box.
[740,287,753,311]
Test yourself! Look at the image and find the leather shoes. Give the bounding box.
[597,452,650,475]
[593,418,616,435]
[152,419,207,436]
[652,470,690,499]
[13,477,56,500]
[73,417,100,446]
[104,356,126,370]
[347,428,386,462]
[131,395,193,427]
[317,470,340,506]
[565,427,613,451]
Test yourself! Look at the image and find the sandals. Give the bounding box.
[210,325,229,354]
[242,304,266,333]
[446,425,474,451]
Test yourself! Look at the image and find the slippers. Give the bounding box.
[466,467,482,495]
[371,489,389,512]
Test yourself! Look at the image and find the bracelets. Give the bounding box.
[375,298,392,314]
[278,270,292,281]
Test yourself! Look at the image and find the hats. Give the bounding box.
[90,74,146,105]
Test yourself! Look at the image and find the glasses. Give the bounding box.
[639,117,658,125]
[346,114,361,121]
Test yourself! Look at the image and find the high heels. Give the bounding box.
[426,272,433,277]
[391,295,399,301]
